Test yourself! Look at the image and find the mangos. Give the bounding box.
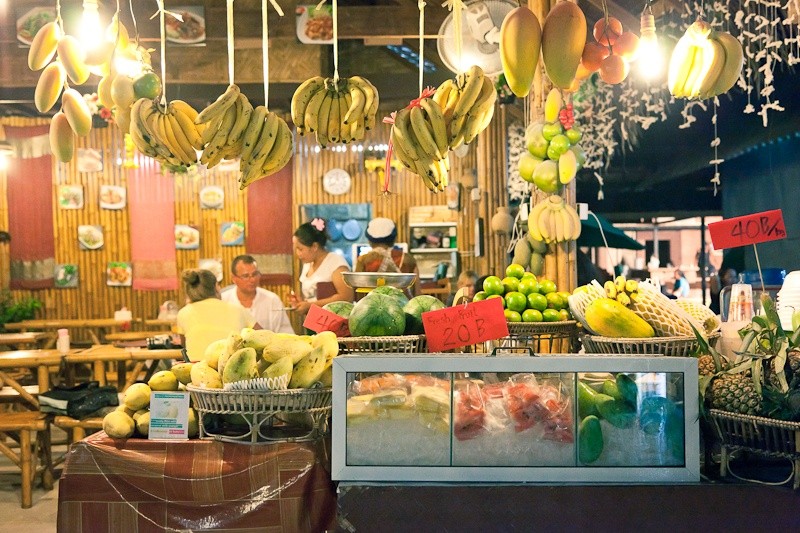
[27,22,160,163]
[512,233,547,275]
[559,150,577,184]
[545,88,561,124]
[500,0,587,95]
[576,379,633,464]
[101,328,340,439]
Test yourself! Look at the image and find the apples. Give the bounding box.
[543,123,582,160]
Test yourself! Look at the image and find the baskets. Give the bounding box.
[579,331,722,358]
[462,319,581,355]
[335,333,429,354]
[706,407,800,490]
[186,383,332,447]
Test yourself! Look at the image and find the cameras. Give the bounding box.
[147,335,172,350]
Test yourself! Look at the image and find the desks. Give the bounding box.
[0,319,187,470]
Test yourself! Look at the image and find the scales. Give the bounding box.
[341,271,417,301]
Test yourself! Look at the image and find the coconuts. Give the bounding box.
[522,131,587,193]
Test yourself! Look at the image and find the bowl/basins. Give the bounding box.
[201,188,223,207]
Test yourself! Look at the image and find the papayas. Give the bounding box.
[572,283,719,338]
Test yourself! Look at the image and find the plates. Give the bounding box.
[200,260,223,283]
[16,6,57,45]
[297,10,334,44]
[59,187,82,209]
[107,266,132,286]
[78,156,103,171]
[175,227,199,248]
[78,226,104,249]
[164,9,205,44]
[223,223,244,245]
[101,186,126,209]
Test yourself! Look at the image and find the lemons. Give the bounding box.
[471,263,573,322]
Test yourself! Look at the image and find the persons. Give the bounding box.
[613,241,716,299]
[353,217,421,304]
[176,268,264,363]
[285,217,353,335]
[444,270,492,309]
[221,255,296,335]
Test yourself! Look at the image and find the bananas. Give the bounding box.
[668,23,743,100]
[127,74,380,190]
[604,276,642,305]
[527,196,582,244]
[388,66,497,194]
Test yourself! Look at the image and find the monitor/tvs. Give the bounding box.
[352,244,408,271]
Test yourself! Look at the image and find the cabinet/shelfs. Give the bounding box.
[407,222,458,282]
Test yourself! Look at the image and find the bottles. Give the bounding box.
[290,290,297,308]
[56,328,70,353]
[411,227,456,247]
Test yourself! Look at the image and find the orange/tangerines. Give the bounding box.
[582,15,642,83]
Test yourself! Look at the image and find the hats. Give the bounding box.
[364,217,397,243]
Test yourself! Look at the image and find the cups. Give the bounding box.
[727,283,755,322]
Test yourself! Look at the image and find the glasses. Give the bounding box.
[233,271,262,282]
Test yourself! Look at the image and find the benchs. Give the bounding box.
[0,369,151,508]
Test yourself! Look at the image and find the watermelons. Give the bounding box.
[320,285,449,336]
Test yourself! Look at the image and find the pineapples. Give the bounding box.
[698,323,800,422]
